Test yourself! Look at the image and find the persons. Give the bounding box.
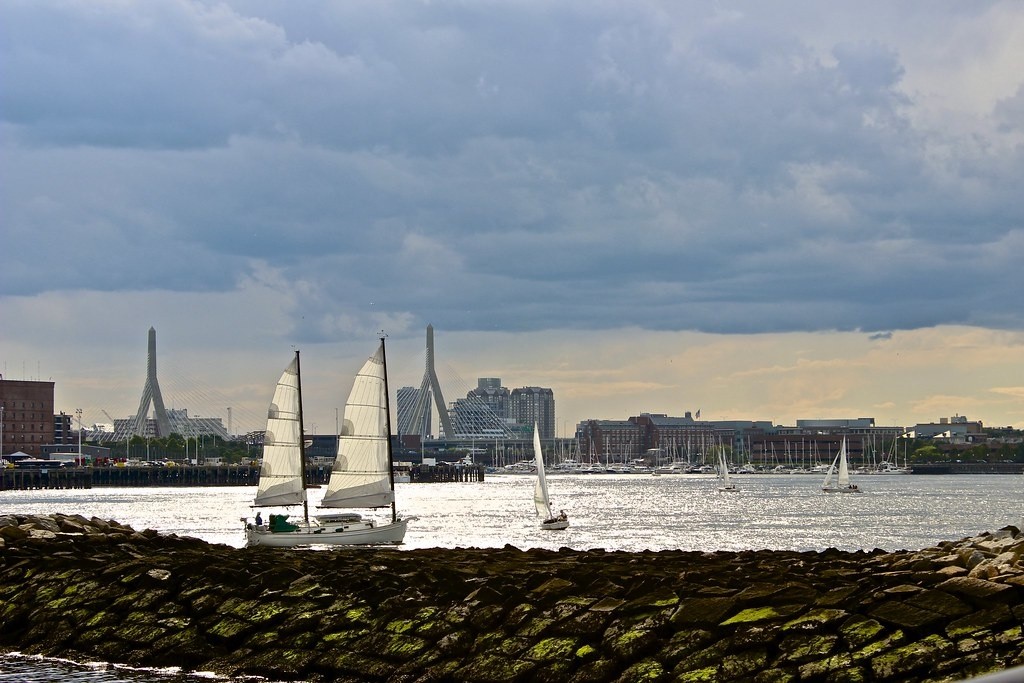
[256,512,262,525]
[559,510,568,521]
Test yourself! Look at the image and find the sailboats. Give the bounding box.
[716,446,738,493]
[239,336,418,546]
[820,434,861,494]
[531,420,570,530]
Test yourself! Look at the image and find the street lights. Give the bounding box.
[76,408,83,466]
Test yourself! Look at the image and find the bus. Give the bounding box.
[14,459,61,469]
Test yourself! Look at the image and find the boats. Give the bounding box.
[485,432,914,476]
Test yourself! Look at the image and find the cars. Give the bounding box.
[143,460,166,467]
